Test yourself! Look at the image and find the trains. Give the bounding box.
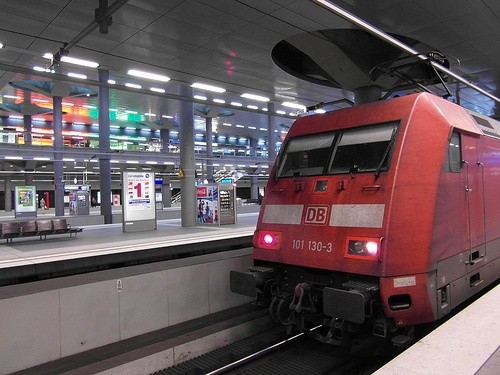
[229,92,500,362]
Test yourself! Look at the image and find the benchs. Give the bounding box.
[0,217,84,245]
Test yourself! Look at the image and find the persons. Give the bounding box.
[40,198,45,214]
[199,200,205,223]
[92,197,96,207]
[205,202,210,223]
